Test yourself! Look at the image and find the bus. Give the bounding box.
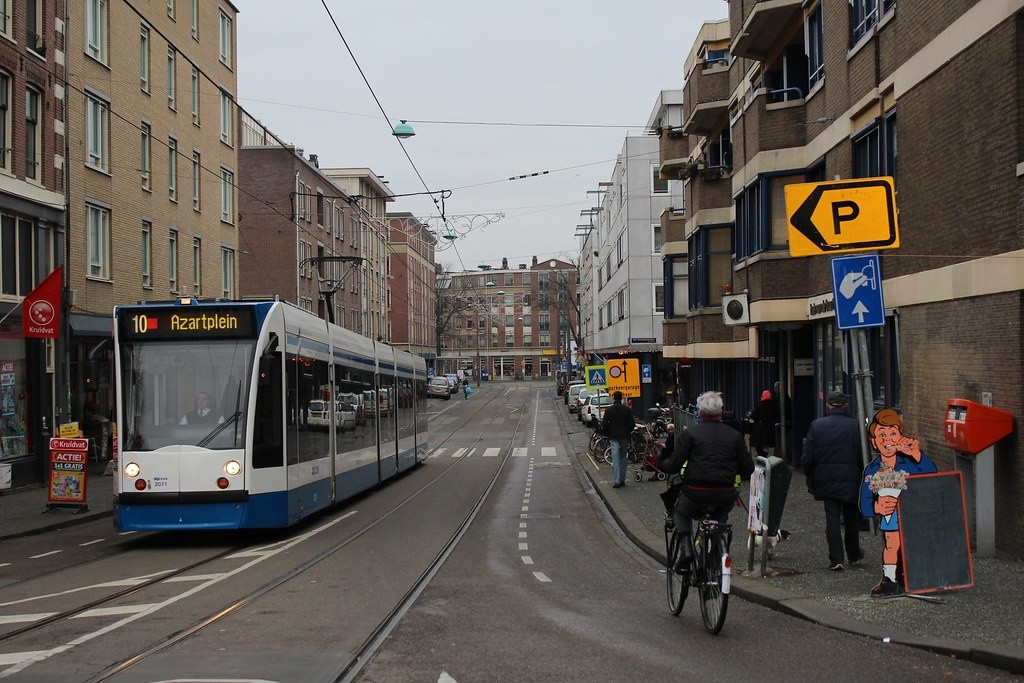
[110,294,430,529]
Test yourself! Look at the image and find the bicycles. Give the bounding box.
[588,413,647,466]
[658,473,734,636]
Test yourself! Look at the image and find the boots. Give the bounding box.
[676,536,695,568]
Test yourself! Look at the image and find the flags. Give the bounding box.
[22,265,62,338]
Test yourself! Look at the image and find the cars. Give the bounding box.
[427,377,451,399]
[448,379,457,393]
[304,384,412,427]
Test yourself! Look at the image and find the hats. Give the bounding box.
[828,391,847,406]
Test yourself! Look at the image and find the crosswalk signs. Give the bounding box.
[589,369,606,384]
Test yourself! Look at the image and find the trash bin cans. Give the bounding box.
[747,455,793,536]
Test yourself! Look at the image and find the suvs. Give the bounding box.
[581,394,615,427]
[574,389,607,421]
[557,370,577,396]
[568,384,587,414]
[443,373,461,392]
[564,380,585,405]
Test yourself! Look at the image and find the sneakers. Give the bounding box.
[847,550,864,563]
[829,562,844,570]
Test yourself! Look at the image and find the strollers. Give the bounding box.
[634,439,666,482]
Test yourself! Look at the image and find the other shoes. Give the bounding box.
[611,482,625,488]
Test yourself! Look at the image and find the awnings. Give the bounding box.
[70,313,114,336]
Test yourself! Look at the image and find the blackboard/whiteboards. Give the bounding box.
[897,471,974,595]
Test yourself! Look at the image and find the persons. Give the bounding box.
[803,391,866,570]
[660,392,755,600]
[180,392,225,425]
[649,424,675,480]
[601,391,635,486]
[463,377,469,393]
[773,382,793,463]
[751,390,778,457]
